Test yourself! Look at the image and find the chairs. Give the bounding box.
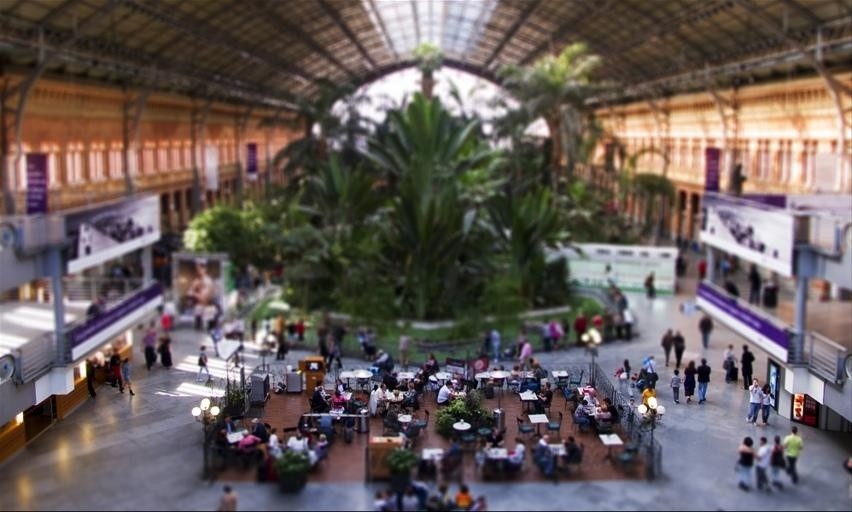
[224,368,647,475]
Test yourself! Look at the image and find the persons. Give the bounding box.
[734,426,805,494]
[82,219,303,399]
[732,162,748,197]
[604,233,779,427]
[478,313,627,479]
[212,308,488,511]
